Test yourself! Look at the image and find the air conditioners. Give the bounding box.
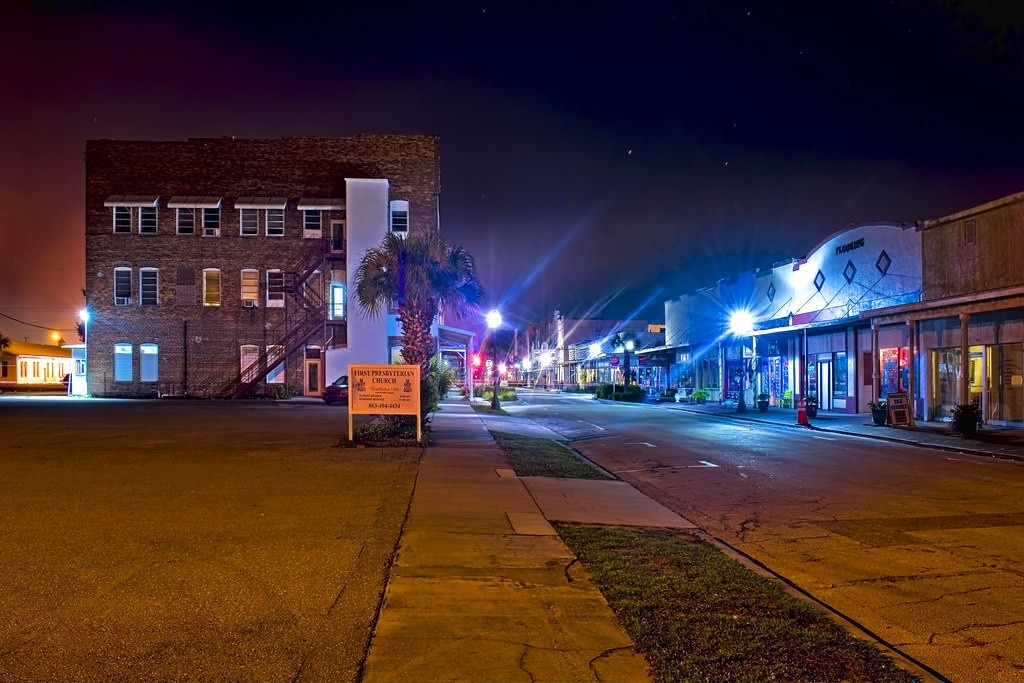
[115,298,128,307]
[244,300,254,307]
[203,228,216,237]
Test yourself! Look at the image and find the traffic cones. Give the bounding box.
[795,399,810,426]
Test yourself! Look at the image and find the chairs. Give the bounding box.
[778,390,792,408]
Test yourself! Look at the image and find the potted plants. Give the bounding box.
[757,393,770,413]
[868,400,887,425]
[951,402,984,437]
[692,389,709,404]
[805,396,818,418]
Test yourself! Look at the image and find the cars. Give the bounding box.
[320,375,349,405]
[63,374,72,385]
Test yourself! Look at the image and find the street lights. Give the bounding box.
[543,355,549,390]
[522,360,530,390]
[593,345,600,386]
[736,311,753,413]
[85,312,90,384]
[626,341,634,391]
[514,365,518,382]
[486,310,503,411]
